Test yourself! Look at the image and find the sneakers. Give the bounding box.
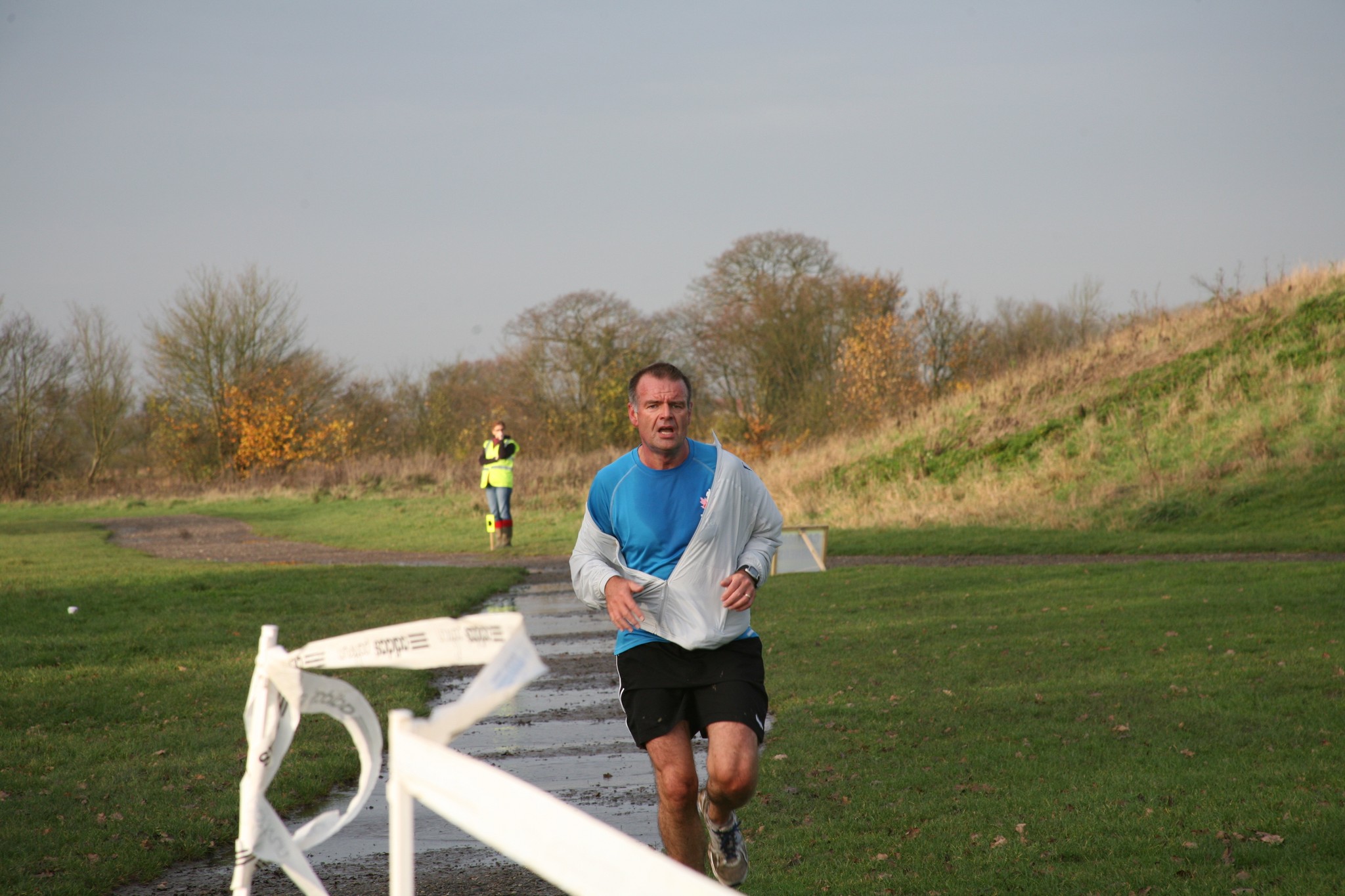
[698,789,749,887]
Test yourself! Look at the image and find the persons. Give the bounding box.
[569,362,783,888]
[478,421,520,549]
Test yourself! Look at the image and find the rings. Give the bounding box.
[743,593,751,598]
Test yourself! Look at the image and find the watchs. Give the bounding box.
[733,564,760,588]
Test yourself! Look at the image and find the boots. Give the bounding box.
[494,519,512,547]
